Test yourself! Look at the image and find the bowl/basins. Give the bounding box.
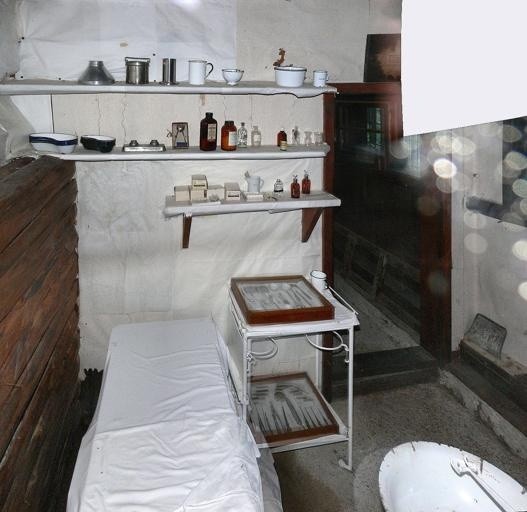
[274,66,306,89]
[222,69,245,86]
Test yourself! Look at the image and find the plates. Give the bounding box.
[27,132,115,154]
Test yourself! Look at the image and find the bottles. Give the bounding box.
[220,120,237,150]
[200,112,218,151]
[238,123,247,149]
[301,175,311,195]
[273,178,284,198]
[251,126,260,146]
[276,128,287,151]
[290,178,300,199]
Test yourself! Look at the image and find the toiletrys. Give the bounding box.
[302,170,311,194]
[290,174,300,199]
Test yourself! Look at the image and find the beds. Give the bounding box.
[68,310,283,511]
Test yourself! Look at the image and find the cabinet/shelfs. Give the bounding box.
[226,275,360,473]
[0,79,342,250]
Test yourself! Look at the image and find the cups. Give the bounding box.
[247,176,264,193]
[303,131,324,149]
[188,60,213,86]
[312,70,328,88]
[309,270,329,293]
[162,58,176,84]
